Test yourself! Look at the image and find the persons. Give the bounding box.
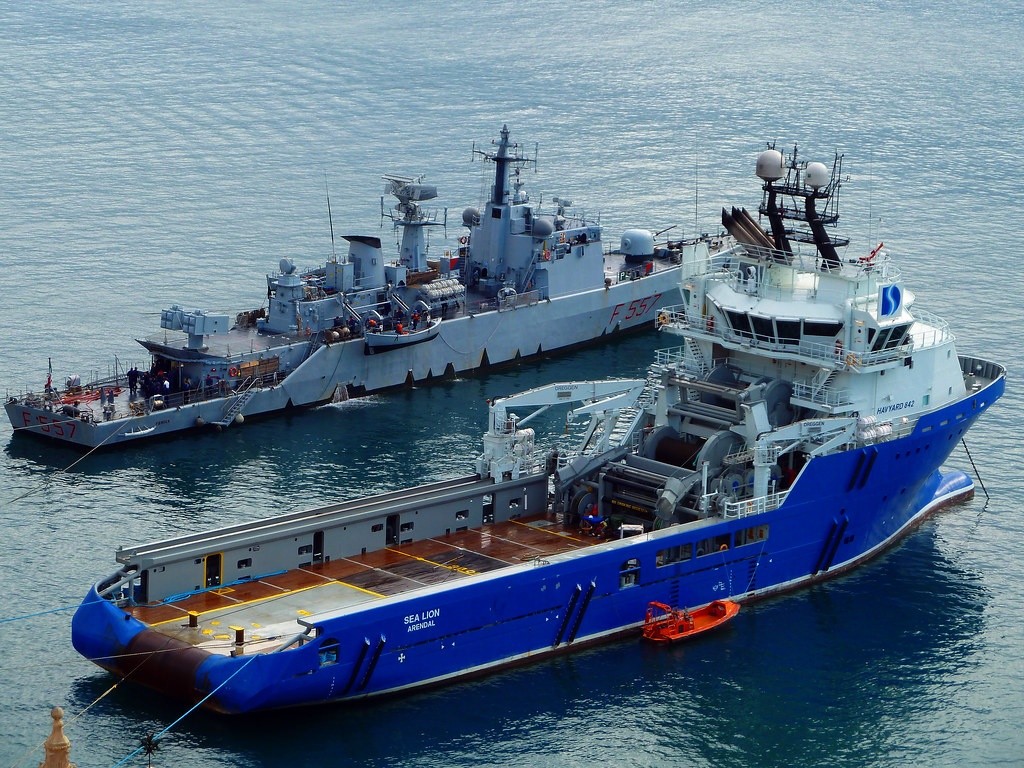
[582,519,595,536]
[474,270,479,284]
[349,316,359,336]
[395,321,403,334]
[369,320,378,334]
[440,294,448,313]
[205,374,213,397]
[127,367,173,408]
[600,520,608,534]
[182,380,190,396]
[334,316,340,327]
[580,230,586,243]
[412,310,419,331]
[645,259,653,274]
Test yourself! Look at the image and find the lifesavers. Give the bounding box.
[658,313,669,323]
[461,236,467,244]
[229,367,237,376]
[846,355,857,367]
[544,250,551,260]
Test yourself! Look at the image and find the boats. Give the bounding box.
[640,597,742,649]
[3,121,743,449]
[69,134,1010,715]
[363,317,443,355]
